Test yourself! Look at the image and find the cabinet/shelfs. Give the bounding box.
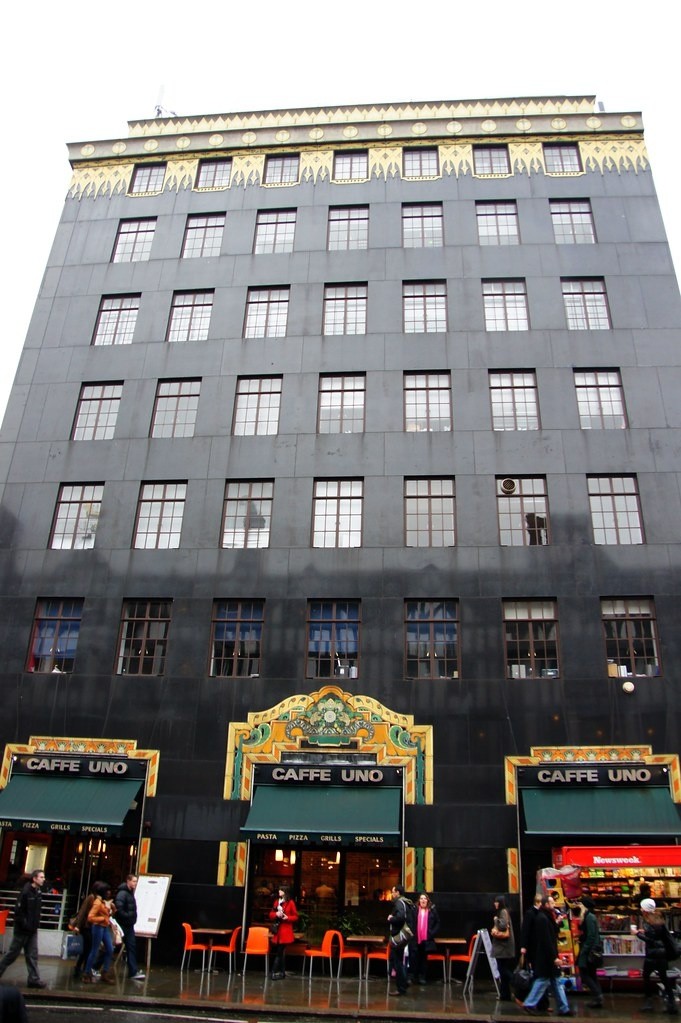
[539,867,681,994]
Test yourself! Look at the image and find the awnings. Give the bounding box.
[0,776,144,840]
[239,784,403,852]
[520,788,681,836]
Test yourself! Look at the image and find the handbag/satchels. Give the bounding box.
[391,923,414,948]
[587,951,604,969]
[108,917,124,945]
[490,909,510,939]
[270,921,280,934]
[62,931,83,960]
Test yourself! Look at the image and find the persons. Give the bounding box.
[407,892,441,986]
[633,883,651,908]
[630,897,678,1016]
[515,897,576,1017]
[253,880,280,908]
[268,885,299,981]
[0,870,67,989]
[488,893,526,1002]
[573,896,605,1007]
[73,880,118,984]
[315,876,336,898]
[387,884,418,997]
[372,888,385,901]
[91,874,146,979]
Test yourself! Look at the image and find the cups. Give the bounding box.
[630,925,638,934]
[55,904,60,913]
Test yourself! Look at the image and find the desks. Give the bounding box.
[346,936,386,979]
[191,928,234,973]
[268,933,303,973]
[435,938,466,981]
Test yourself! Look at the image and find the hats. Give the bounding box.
[640,898,656,912]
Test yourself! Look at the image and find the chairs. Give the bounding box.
[181,920,208,974]
[449,934,477,983]
[335,931,363,979]
[366,942,391,979]
[303,930,336,978]
[208,926,242,976]
[243,927,270,977]
[427,954,447,983]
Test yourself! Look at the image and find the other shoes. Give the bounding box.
[515,998,527,1011]
[27,978,47,990]
[73,970,115,983]
[130,970,146,978]
[557,1008,572,1017]
[271,972,287,980]
[584,999,604,1009]
[638,1005,656,1013]
[389,990,401,996]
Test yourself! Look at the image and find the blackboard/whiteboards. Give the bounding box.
[133,873,172,938]
[466,928,500,978]
